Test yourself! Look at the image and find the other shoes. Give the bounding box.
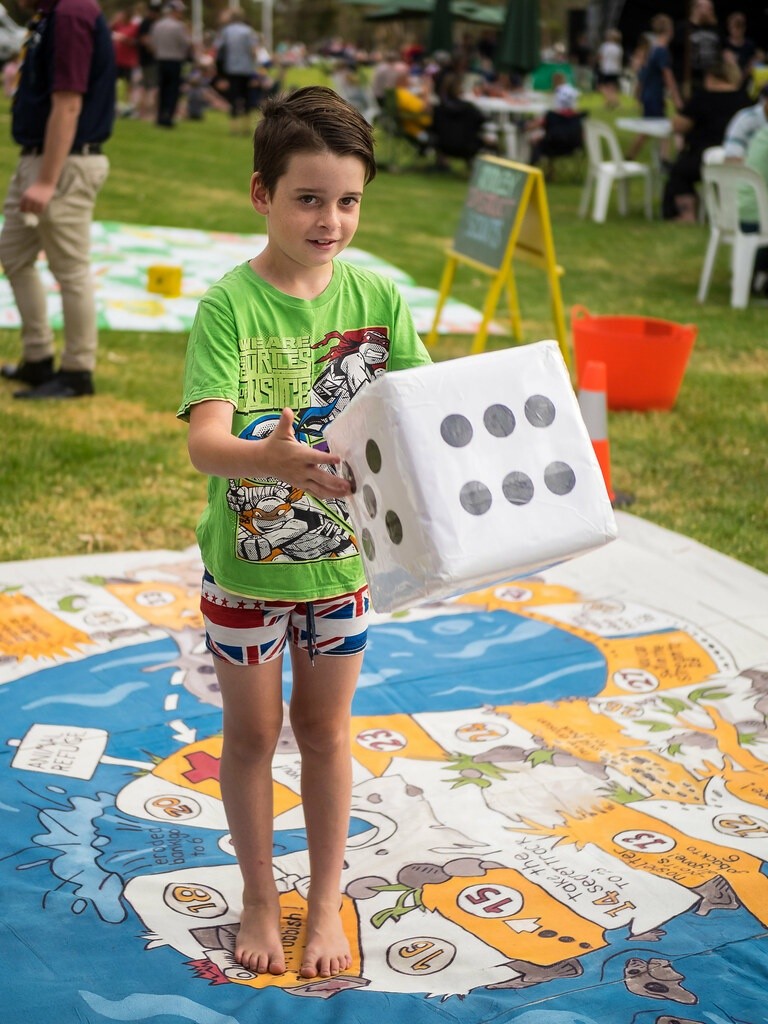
[2,356,53,386]
[15,373,93,398]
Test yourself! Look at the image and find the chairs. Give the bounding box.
[578,118,653,223]
[698,145,768,310]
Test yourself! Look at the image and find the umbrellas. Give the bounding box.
[365,0,505,24]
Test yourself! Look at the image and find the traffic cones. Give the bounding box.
[576,359,617,502]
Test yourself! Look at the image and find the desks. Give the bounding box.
[614,116,673,167]
[477,99,551,160]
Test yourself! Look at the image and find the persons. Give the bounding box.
[177,88,432,978]
[107,0,768,296]
[0,0,116,400]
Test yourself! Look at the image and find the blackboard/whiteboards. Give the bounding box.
[444,155,535,276]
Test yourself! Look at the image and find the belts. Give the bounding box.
[20,145,101,155]
